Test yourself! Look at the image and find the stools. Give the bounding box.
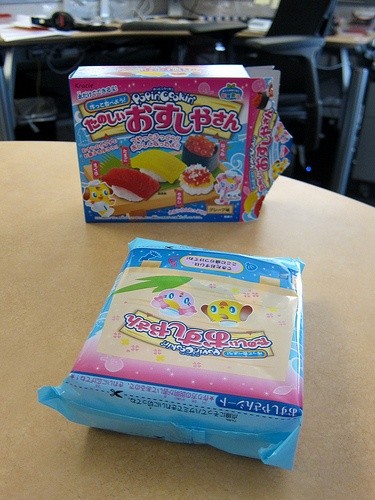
[243,33,375,151]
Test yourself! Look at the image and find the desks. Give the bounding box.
[0,141,375,500]
[0,19,375,129]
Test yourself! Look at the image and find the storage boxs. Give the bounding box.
[68,64,294,223]
